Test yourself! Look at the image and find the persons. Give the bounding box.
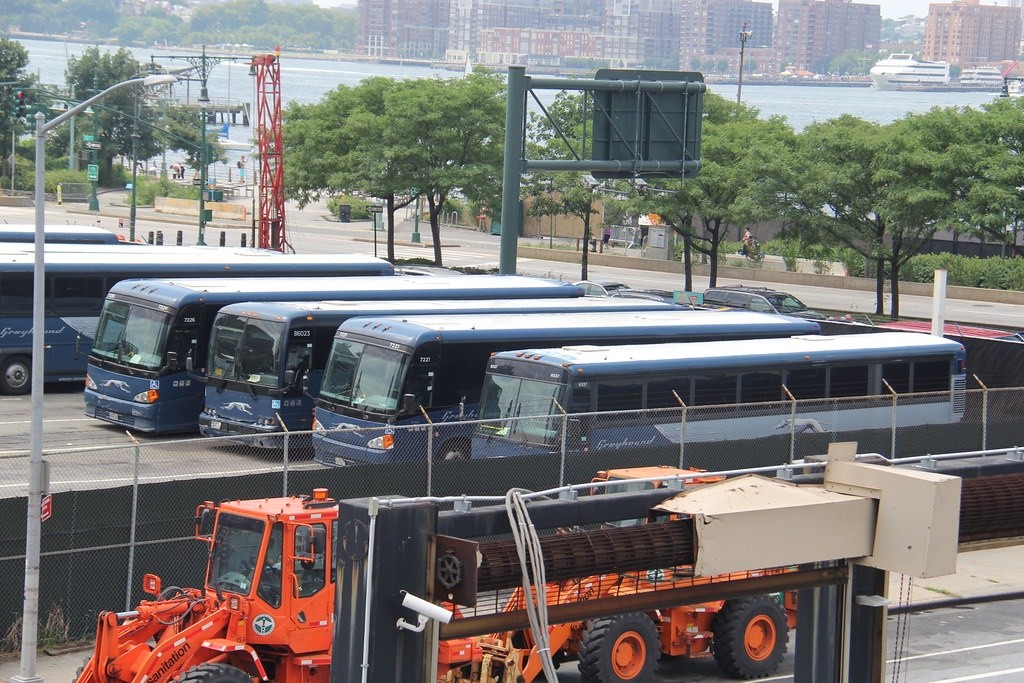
[193,170,199,178]
[170,163,185,179]
[238,155,248,183]
[738,227,756,257]
[603,225,611,250]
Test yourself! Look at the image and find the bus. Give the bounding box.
[0,237,395,396]
[75,270,587,437]
[299,311,821,466]
[186,294,689,450]
[0,221,119,245]
[462,326,968,457]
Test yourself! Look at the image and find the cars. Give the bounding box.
[573,278,673,304]
[394,264,467,276]
[880,320,1024,345]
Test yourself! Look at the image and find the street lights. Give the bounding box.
[147,44,257,247]
[732,21,755,124]
[128,73,210,242]
[7,74,180,683]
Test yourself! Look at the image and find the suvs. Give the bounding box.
[701,283,829,321]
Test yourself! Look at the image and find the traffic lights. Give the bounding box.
[11,90,25,118]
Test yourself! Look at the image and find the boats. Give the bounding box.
[869,53,1004,91]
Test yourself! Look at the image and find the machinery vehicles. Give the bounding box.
[74,465,802,683]
